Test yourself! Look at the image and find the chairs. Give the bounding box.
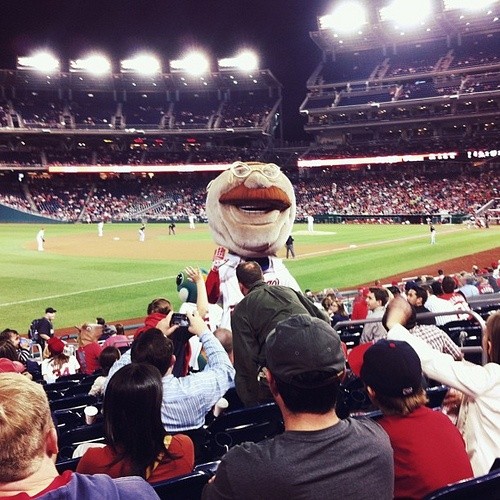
[38,301,500,500]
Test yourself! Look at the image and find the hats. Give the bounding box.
[463,273,474,279]
[265,313,346,388]
[347,339,423,400]
[491,261,497,267]
[0,358,24,373]
[479,269,488,276]
[46,307,56,314]
[46,337,65,354]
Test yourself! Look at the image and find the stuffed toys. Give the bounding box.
[205,159,302,332]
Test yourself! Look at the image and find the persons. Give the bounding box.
[0,98,500,500]
[200,314,394,500]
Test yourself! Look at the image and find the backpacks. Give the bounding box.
[28,319,52,344]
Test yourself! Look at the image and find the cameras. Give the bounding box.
[171,314,190,327]
